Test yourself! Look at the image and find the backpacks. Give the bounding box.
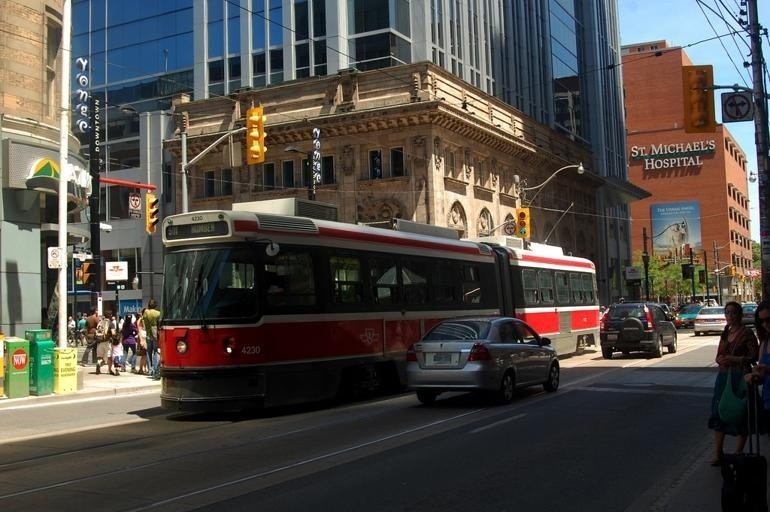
[95,320,109,340]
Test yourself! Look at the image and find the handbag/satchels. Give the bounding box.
[718,368,747,423]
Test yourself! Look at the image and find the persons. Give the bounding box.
[704,300,758,466]
[743,300,769,444]
[618,297,625,305]
[599,303,612,330]
[67,315,76,344]
[75,297,160,381]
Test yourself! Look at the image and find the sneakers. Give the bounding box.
[80,360,160,377]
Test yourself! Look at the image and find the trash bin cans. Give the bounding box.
[6,330,55,399]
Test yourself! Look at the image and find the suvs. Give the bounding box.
[599,301,678,358]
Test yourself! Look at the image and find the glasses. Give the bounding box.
[755,317,768,322]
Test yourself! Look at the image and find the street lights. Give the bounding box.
[284,147,313,200]
[713,238,742,307]
[87,95,137,316]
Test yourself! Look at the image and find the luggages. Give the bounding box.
[721,453,769,511]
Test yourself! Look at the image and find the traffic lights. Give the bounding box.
[515,207,531,238]
[245,105,268,166]
[144,192,160,235]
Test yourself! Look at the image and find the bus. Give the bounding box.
[158,196,600,413]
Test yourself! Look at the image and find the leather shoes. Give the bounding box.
[708,450,723,464]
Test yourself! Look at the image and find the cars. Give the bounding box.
[405,315,561,405]
[659,298,759,337]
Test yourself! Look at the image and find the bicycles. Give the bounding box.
[68,329,83,346]
